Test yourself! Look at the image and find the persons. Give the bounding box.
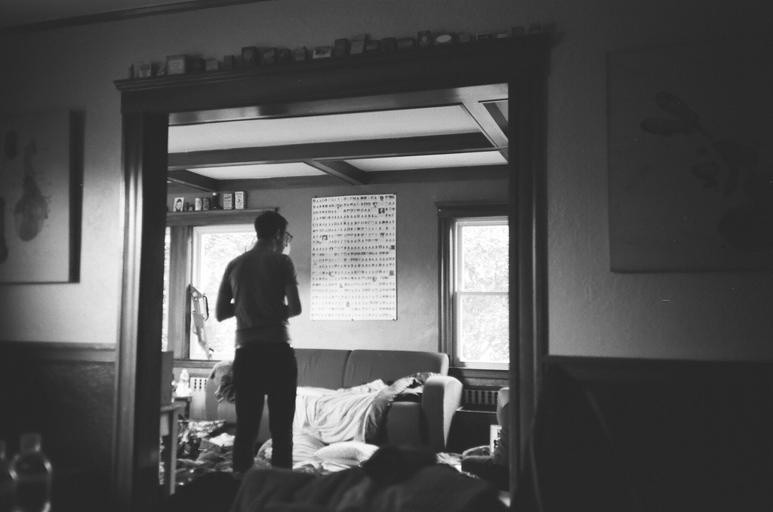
[215,211,302,480]
[174,199,182,212]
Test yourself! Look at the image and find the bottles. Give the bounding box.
[194,192,221,211]
[10,429,53,512]
[179,368,189,396]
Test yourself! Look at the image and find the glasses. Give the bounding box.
[280,230,293,243]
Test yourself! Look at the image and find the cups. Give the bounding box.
[490,424,502,456]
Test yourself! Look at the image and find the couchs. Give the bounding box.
[206,343,468,463]
